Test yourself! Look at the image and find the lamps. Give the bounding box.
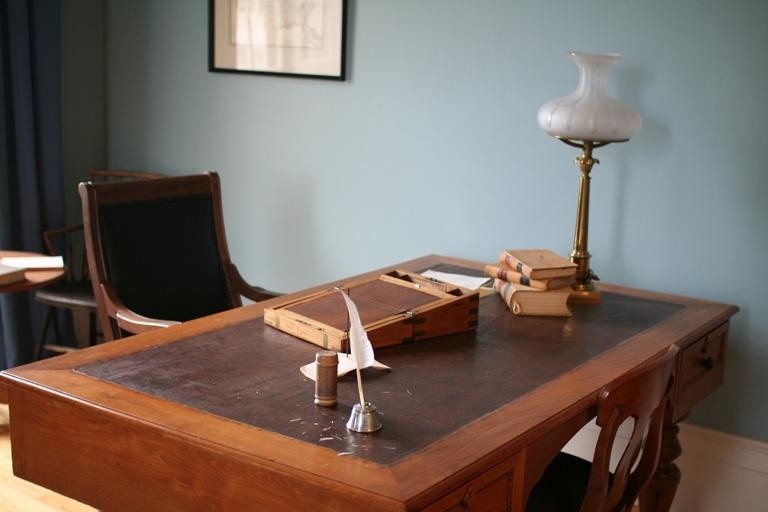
[536,50,641,305]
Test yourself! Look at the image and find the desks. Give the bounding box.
[0,253,739,511]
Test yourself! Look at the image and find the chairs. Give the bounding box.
[69,138,280,345]
[33,165,170,365]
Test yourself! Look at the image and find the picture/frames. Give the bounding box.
[207,2,349,80]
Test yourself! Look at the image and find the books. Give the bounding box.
[504,249,579,280]
[491,278,576,318]
[484,262,577,291]
[2,264,27,288]
